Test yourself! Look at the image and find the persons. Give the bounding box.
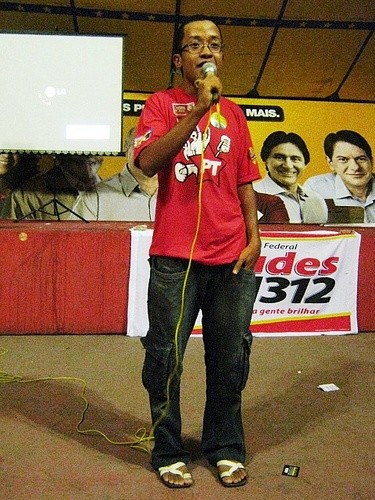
[0,126,164,224]
[133,15,262,488]
[250,128,375,224]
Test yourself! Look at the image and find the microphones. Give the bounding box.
[201,62,220,103]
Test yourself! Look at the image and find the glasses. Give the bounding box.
[179,39,225,53]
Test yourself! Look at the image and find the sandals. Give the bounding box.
[216,459,247,487]
[158,462,193,488]
